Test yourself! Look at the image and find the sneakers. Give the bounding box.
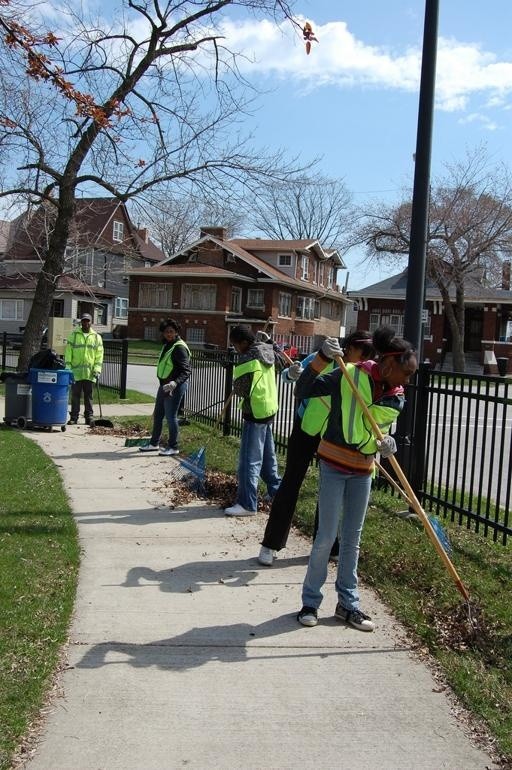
[68,421,76,425]
[259,546,273,566]
[225,504,257,516]
[159,447,179,456]
[297,606,318,627]
[334,603,374,631]
[85,417,93,423]
[139,444,159,452]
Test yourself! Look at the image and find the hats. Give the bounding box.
[81,313,91,322]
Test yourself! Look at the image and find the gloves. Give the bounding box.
[288,361,303,380]
[376,435,397,459]
[93,371,100,379]
[163,381,177,397]
[322,338,344,359]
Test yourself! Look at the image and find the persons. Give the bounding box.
[296,330,419,632]
[139,319,193,457]
[260,331,373,565]
[66,313,103,425]
[225,323,281,516]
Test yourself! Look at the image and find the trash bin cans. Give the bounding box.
[30,368,75,432]
[0,371,33,430]
